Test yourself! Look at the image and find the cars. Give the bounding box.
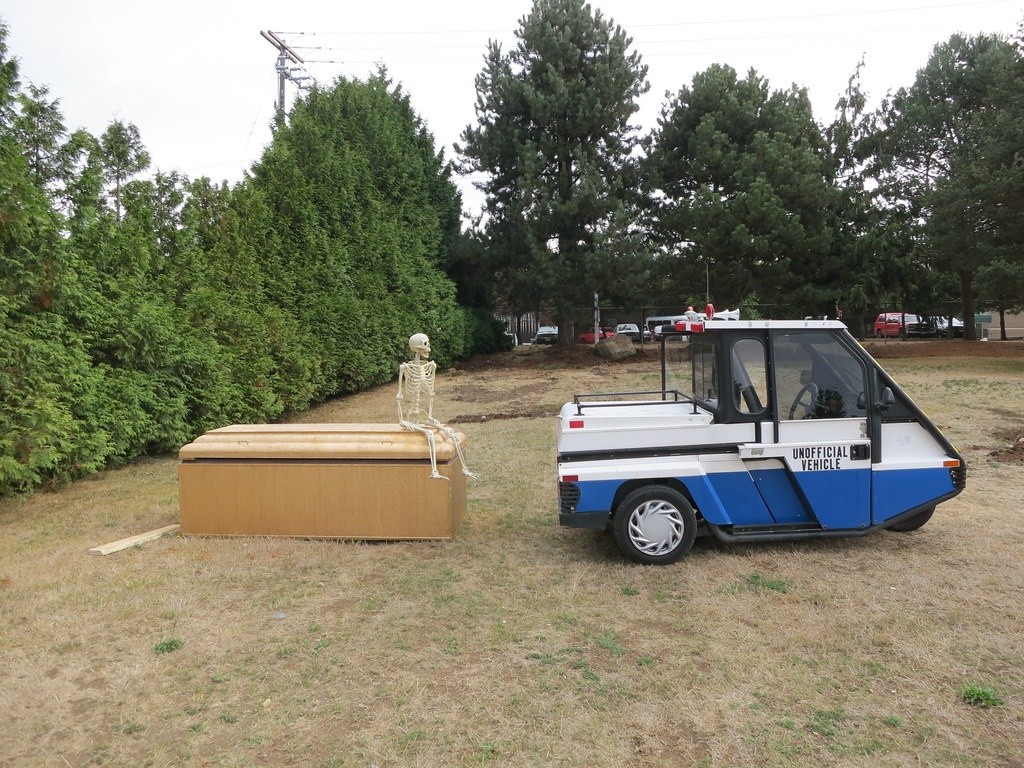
[614,324,640,342]
[930,316,964,340]
[536,326,558,344]
[577,327,614,344]
[654,325,664,341]
[643,324,652,342]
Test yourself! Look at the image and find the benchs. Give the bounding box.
[177,422,469,542]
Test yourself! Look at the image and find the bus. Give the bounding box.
[646,316,690,332]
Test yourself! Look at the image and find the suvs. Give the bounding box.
[874,313,930,338]
